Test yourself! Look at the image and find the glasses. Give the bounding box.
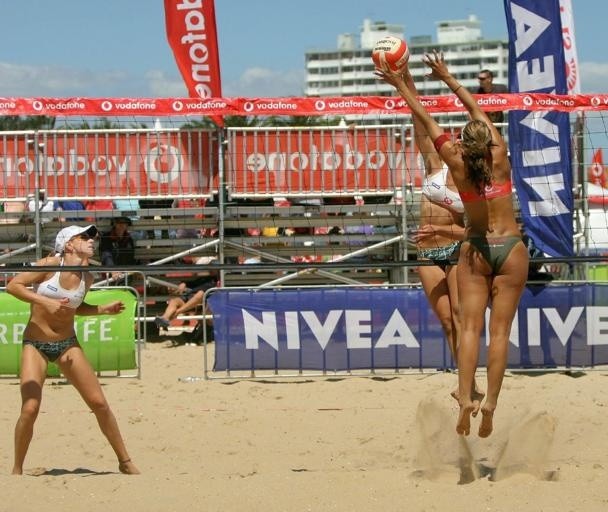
[477,76,486,80]
[70,234,94,241]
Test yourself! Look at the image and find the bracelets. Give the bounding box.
[453,86,461,93]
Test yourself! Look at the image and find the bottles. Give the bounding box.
[177,376,206,384]
[162,339,177,348]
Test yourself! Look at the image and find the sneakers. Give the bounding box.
[155,316,169,328]
[156,311,175,321]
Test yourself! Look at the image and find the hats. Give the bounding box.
[54,225,97,256]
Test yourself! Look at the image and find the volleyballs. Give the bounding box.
[373,35,409,72]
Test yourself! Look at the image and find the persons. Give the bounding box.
[469,70,504,135]
[6,225,141,476]
[402,63,485,417]
[373,48,530,438]
[1,143,396,326]
